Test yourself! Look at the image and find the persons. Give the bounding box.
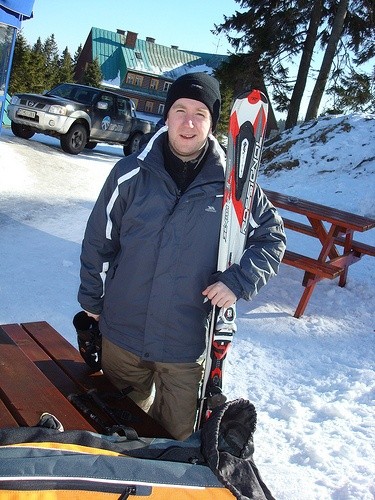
[77,72,286,444]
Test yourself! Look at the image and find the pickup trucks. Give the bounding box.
[8,82,156,156]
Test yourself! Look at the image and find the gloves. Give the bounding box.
[72,311,102,373]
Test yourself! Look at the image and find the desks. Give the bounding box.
[261,188,375,320]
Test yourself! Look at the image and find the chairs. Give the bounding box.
[78,94,88,100]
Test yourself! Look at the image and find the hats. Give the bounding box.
[163,73,221,134]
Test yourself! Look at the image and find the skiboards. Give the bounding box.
[195,88,270,431]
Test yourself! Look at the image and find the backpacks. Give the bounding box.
[0,398,277,499]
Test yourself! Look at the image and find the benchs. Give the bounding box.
[277,247,344,318]
[1,321,178,444]
[281,215,374,288]
